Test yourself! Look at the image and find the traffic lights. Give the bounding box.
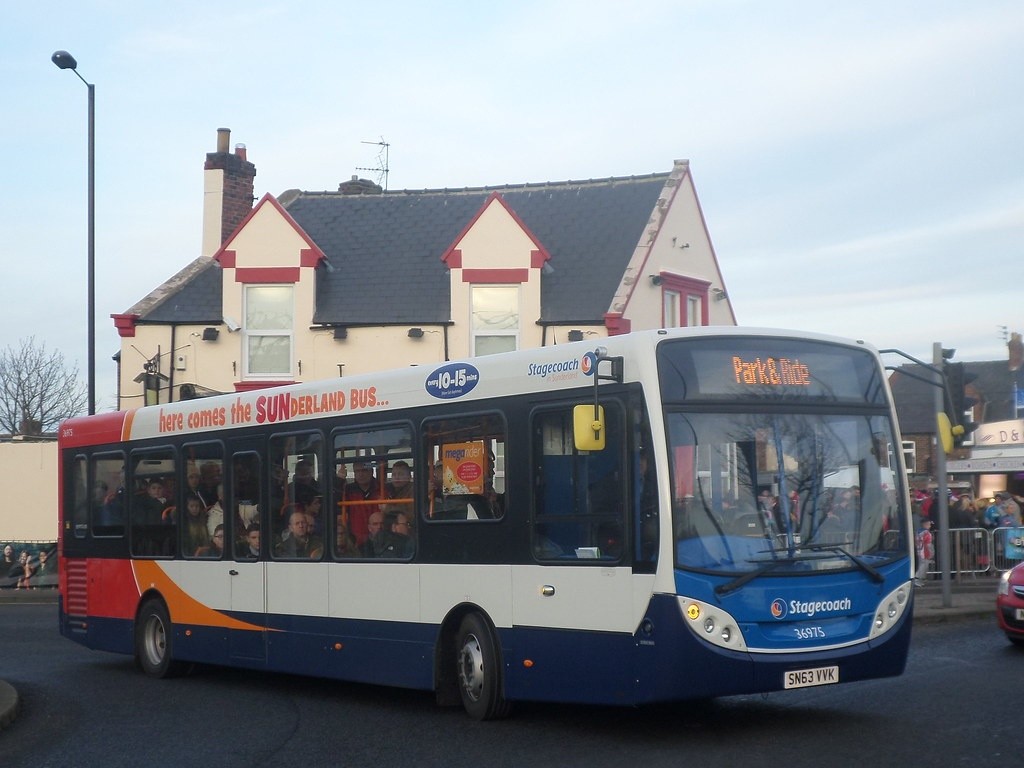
[942,346,979,447]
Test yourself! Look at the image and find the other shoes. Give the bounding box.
[915,579,924,586]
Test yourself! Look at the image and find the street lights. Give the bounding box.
[50,50,97,420]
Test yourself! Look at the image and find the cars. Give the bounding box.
[996,561,1024,648]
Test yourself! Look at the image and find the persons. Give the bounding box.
[706,483,1024,580]
[587,437,695,557]
[0,545,51,586]
[94,458,442,559]
[914,518,932,586]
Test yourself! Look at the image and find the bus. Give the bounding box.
[54,325,966,724]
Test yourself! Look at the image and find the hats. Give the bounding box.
[955,500,968,512]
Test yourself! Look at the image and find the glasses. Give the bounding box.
[397,522,411,526]
[214,535,223,538]
